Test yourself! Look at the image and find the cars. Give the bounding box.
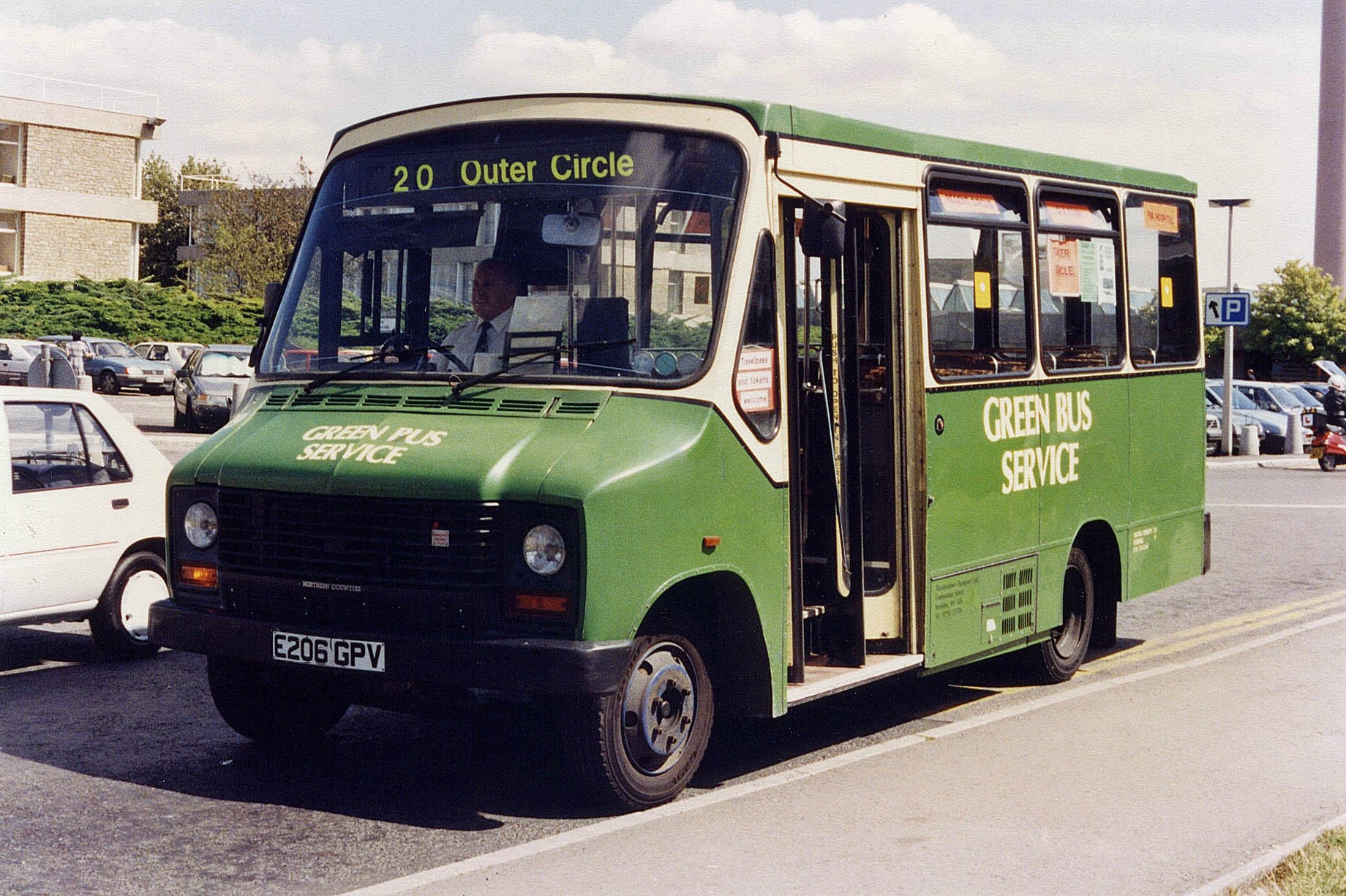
[1206,360,1346,457]
[172,347,291,433]
[283,345,437,374]
[126,341,205,369]
[32,334,177,396]
[0,385,176,664]
[0,338,68,387]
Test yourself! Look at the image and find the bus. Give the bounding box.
[164,88,1212,815]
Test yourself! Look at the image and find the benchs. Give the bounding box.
[11,463,126,489]
[796,342,1157,394]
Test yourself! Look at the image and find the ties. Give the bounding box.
[470,322,493,373]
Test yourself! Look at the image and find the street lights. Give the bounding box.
[1208,199,1255,456]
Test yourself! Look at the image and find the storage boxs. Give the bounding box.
[1301,407,1328,427]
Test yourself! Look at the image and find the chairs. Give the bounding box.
[572,297,631,374]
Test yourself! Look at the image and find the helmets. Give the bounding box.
[1327,374,1346,391]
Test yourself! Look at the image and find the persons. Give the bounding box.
[1321,374,1346,431]
[428,255,555,376]
[65,331,96,376]
[1248,369,1257,381]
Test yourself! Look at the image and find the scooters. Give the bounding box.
[1300,406,1346,472]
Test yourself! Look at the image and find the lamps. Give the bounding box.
[147,117,166,126]
